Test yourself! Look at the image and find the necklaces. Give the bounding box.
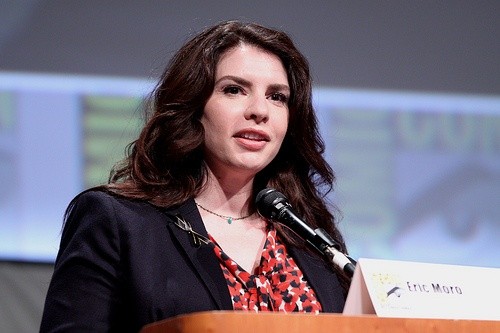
[193,200,260,223]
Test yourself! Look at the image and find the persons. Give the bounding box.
[39,20,357,333]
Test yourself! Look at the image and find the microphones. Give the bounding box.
[256,188,357,278]
